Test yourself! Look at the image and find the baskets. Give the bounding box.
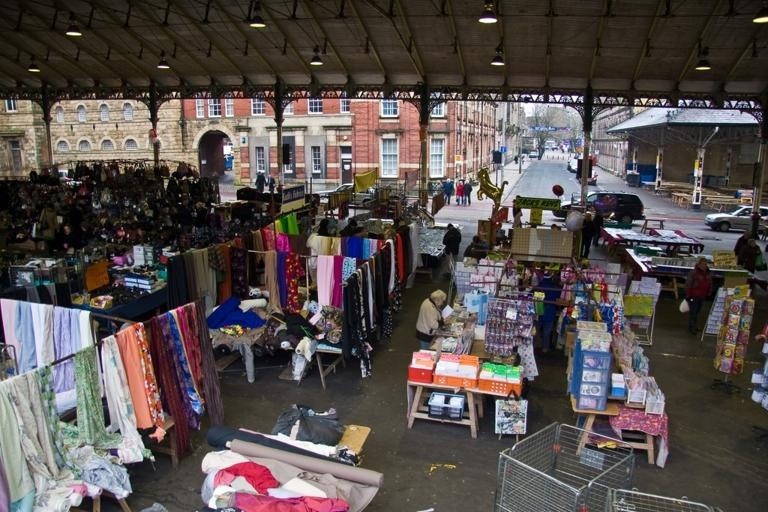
[427,391,465,422]
[478,370,523,396]
[432,369,478,388]
[408,368,432,383]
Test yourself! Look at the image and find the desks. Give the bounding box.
[643,180,751,212]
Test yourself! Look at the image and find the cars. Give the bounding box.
[528,151,539,159]
[568,158,578,172]
[704,204,768,233]
[580,170,598,185]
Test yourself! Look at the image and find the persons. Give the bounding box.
[512,199,522,226]
[580,204,604,259]
[742,239,761,274]
[464,236,487,257]
[432,178,473,206]
[265,175,276,194]
[684,258,713,335]
[416,290,452,350]
[255,172,267,192]
[734,230,753,265]
[442,223,462,277]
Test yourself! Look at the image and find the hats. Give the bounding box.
[544,271,553,278]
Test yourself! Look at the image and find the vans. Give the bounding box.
[552,192,646,226]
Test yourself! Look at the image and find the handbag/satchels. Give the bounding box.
[41,221,48,230]
[755,254,765,266]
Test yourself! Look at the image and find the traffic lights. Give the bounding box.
[493,151,502,163]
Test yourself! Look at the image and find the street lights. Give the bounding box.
[517,128,526,175]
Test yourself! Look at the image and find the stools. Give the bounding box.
[641,218,665,234]
